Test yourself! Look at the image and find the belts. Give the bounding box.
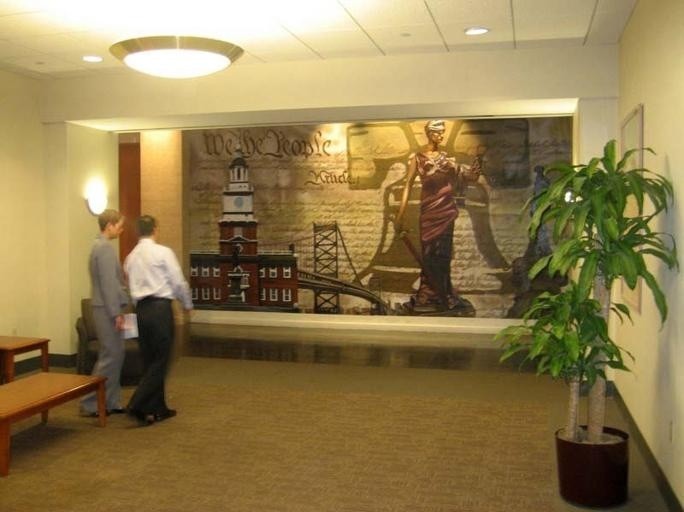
[141,295,171,302]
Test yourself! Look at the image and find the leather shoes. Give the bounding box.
[78,404,125,417]
[122,402,177,426]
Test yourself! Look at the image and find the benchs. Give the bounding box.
[75,298,144,385]
[1,371,109,478]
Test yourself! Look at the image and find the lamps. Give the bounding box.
[108,34,243,79]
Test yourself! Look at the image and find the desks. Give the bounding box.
[0,335,51,383]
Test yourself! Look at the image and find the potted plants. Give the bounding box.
[492,139,681,510]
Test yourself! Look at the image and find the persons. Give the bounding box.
[76,210,129,413]
[395,120,485,309]
[119,216,194,420]
